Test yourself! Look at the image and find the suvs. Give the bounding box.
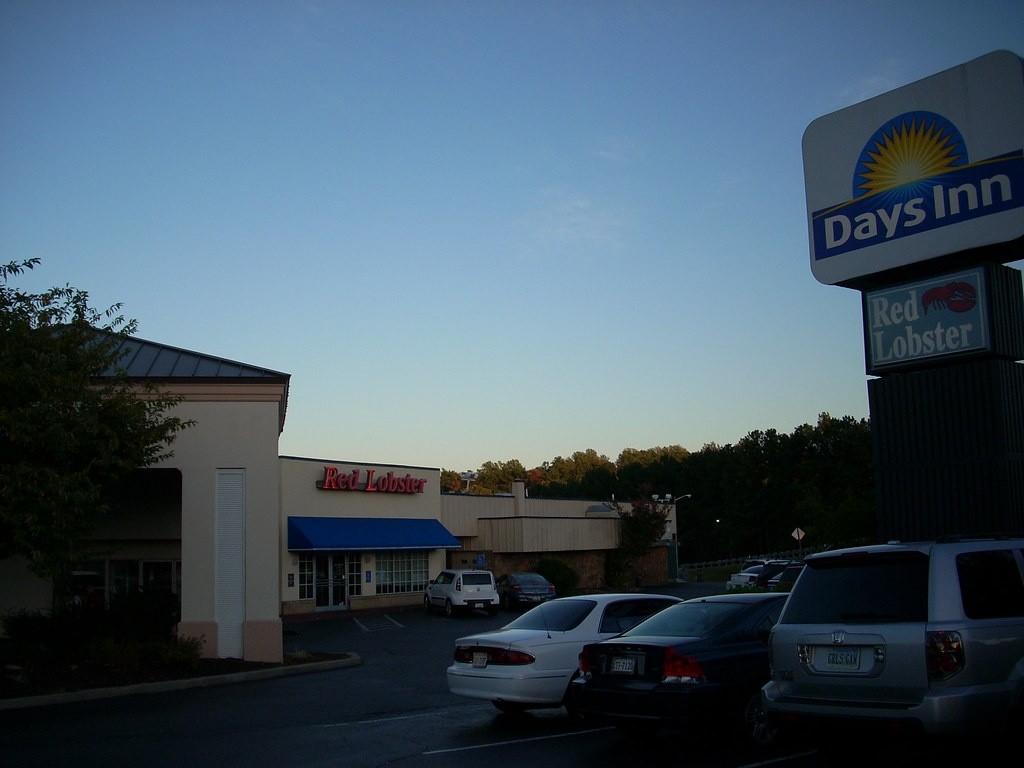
[761,531,1024,740]
[757,563,787,587]
[419,566,501,619]
[742,559,791,573]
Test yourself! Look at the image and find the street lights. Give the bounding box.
[716,517,733,562]
[674,494,692,581]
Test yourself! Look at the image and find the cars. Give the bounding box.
[495,572,556,611]
[725,564,765,590]
[446,593,685,719]
[768,566,805,591]
[572,592,793,732]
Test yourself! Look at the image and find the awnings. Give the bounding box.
[287,517,464,553]
